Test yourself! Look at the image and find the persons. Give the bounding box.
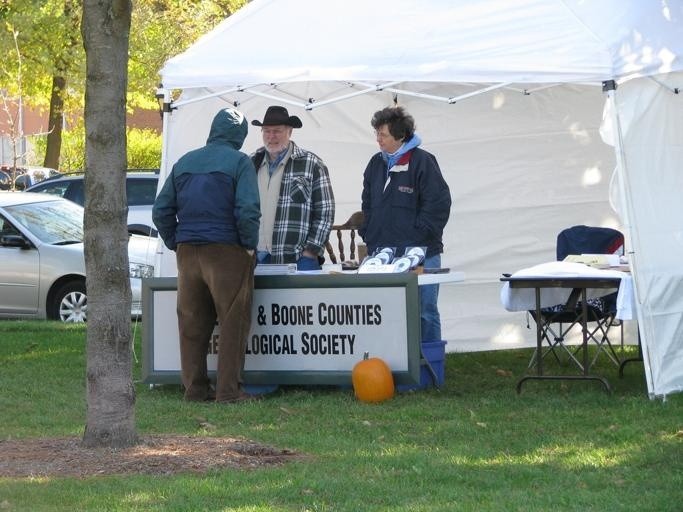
[249,106,335,271]
[358,106,452,342]
[152,108,262,403]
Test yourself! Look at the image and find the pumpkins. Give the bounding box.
[351,351,394,402]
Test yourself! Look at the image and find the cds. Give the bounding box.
[363,247,425,272]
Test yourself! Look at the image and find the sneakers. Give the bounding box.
[206,386,261,402]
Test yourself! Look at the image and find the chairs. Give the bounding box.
[324,210,368,263]
[528,225,625,372]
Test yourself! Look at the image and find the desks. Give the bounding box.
[508,268,645,396]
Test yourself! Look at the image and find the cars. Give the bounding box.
[0,164,159,323]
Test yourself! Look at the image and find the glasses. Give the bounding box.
[260,127,286,136]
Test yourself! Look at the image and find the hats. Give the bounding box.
[251,106,302,128]
